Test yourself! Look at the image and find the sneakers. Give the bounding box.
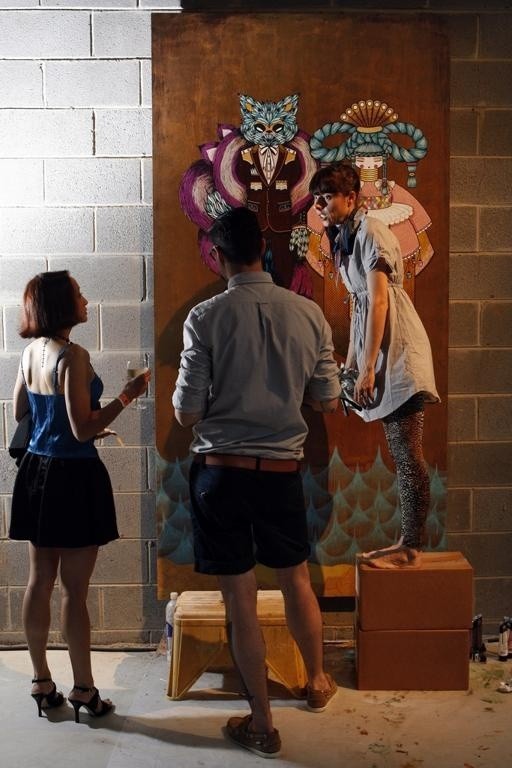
[226,714,281,758]
[356,545,423,569]
[306,672,338,712]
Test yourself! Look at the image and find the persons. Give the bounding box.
[309,164,441,570]
[172,207,343,758]
[8,270,151,722]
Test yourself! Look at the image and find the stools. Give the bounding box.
[166,588,312,701]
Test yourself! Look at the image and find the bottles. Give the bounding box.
[165,591,178,663]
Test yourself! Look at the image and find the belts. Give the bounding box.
[193,453,305,472]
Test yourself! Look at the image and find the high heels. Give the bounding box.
[68,685,112,723]
[31,679,64,717]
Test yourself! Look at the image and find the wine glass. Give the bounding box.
[126,359,147,411]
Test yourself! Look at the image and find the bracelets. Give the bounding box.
[117,387,136,409]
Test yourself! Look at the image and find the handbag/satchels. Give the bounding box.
[8,415,36,465]
